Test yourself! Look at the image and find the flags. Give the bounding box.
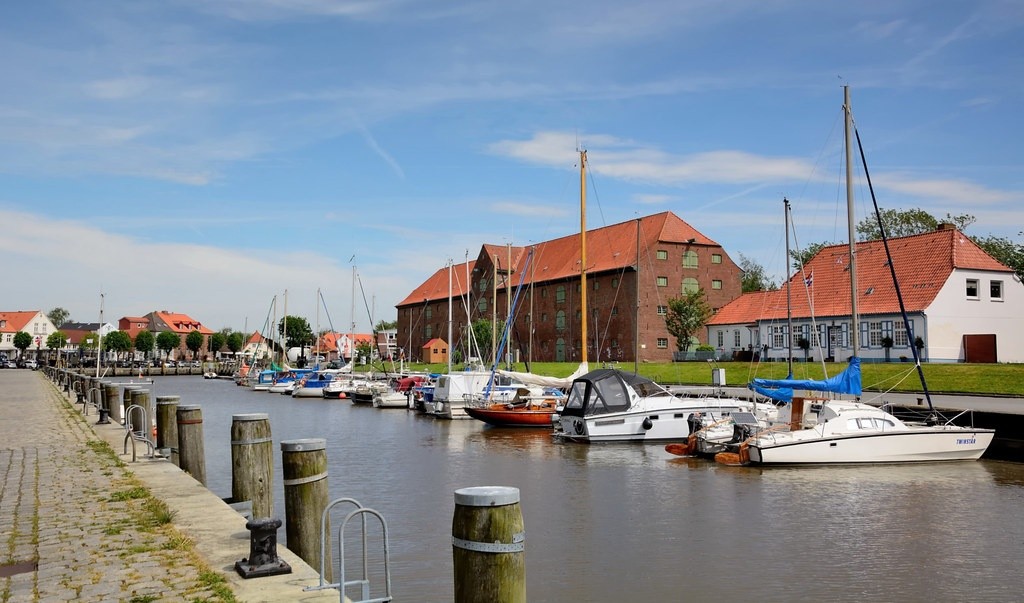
[802,271,814,288]
[387,354,392,363]
[837,253,845,264]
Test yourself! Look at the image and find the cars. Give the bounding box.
[23,359,40,370]
[310,356,326,363]
[65,359,201,368]
[4,361,16,368]
[330,359,345,369]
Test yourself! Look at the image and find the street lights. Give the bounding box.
[96,293,105,377]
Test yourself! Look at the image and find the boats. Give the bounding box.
[553,369,779,442]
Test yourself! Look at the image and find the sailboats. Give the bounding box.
[236,243,536,418]
[463,149,604,425]
[204,335,217,378]
[689,197,823,451]
[741,84,996,462]
